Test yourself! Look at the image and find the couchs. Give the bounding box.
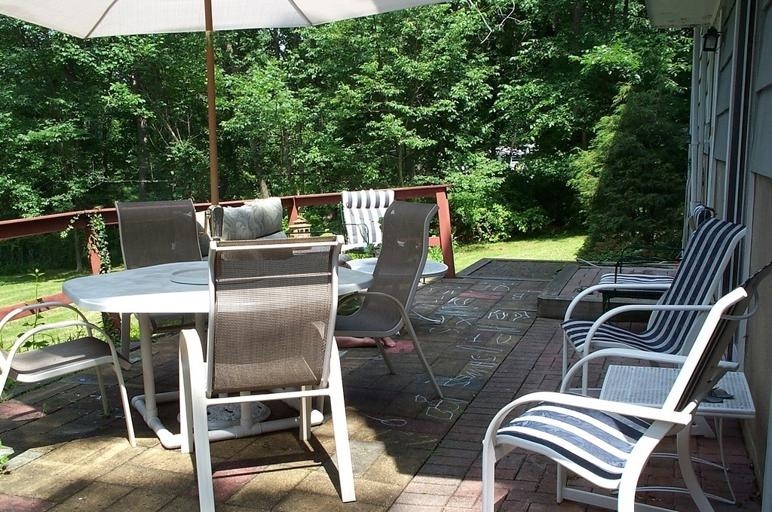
[206,198,352,267]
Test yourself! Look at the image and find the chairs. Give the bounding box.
[559,219,748,395]
[0,301,136,448]
[340,191,396,247]
[599,206,715,321]
[178,234,357,512]
[333,201,445,399]
[482,286,771,512]
[114,198,206,365]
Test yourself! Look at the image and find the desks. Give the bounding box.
[61,261,374,451]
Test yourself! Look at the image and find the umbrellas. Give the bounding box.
[0,0,452,205]
